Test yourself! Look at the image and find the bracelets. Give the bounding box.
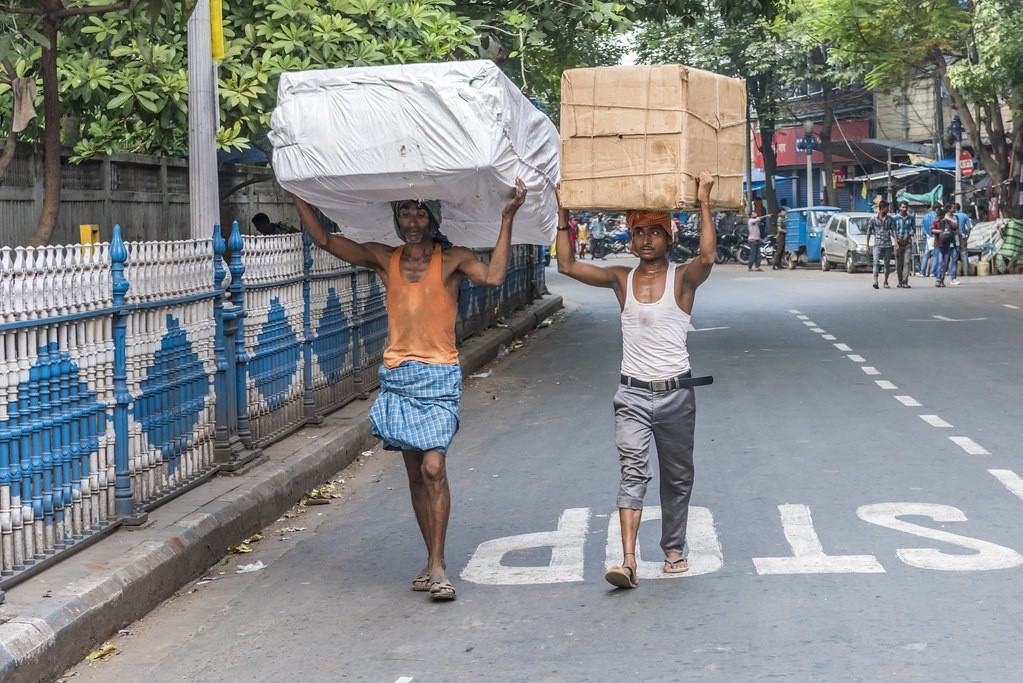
[557,224,570,230]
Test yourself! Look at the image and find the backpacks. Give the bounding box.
[939,220,959,254]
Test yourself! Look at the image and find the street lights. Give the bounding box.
[797,119,820,207]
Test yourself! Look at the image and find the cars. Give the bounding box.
[819,211,896,273]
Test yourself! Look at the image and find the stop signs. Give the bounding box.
[959,151,974,177]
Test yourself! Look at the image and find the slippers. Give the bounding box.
[663,558,689,572]
[605,566,638,587]
[411,567,430,590]
[428,575,456,599]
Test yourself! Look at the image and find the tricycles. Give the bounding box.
[784,206,842,270]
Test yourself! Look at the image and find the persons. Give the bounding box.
[252,212,290,234]
[556,171,717,588]
[867,200,989,289]
[292,177,527,600]
[551,198,790,271]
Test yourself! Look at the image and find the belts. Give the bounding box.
[621,369,714,389]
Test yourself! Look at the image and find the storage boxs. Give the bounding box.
[560,64,748,210]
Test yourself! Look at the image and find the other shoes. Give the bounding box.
[883,282,890,288]
[773,265,787,270]
[748,268,754,272]
[873,282,879,289]
[950,279,961,285]
[756,268,763,272]
[897,281,910,288]
[935,282,945,287]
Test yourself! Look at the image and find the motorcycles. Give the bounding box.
[715,221,748,265]
[670,229,700,263]
[735,232,777,264]
[591,227,632,259]
